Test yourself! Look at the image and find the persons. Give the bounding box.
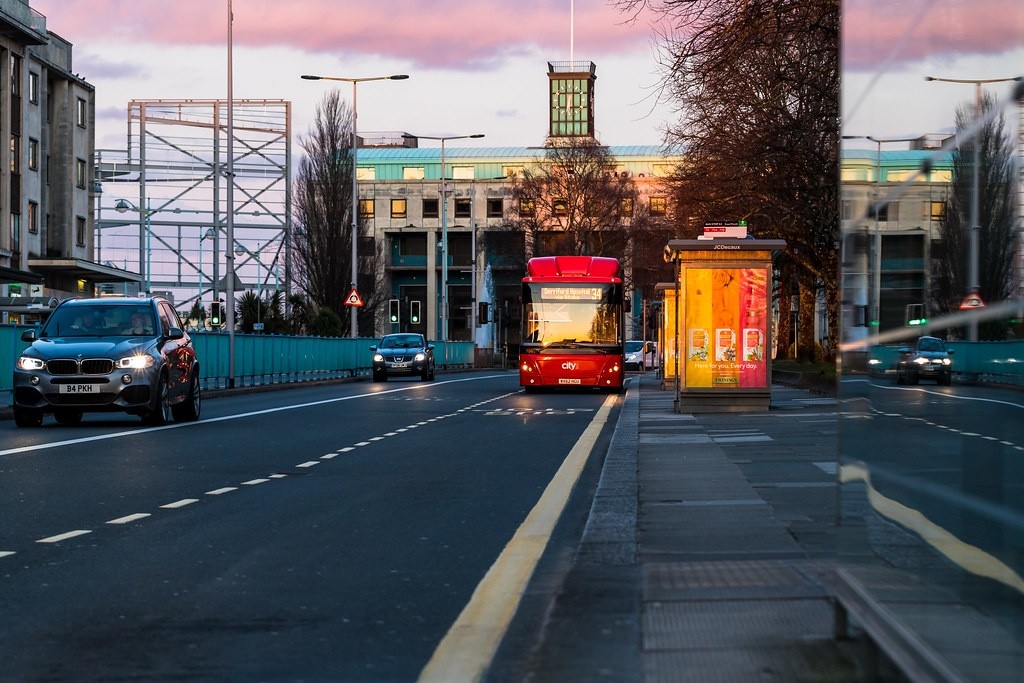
[130,311,151,335]
[71,311,97,335]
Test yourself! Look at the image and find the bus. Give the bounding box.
[520,256,632,390]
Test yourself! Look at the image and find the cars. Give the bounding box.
[624,338,660,371]
[371,333,437,381]
[896,335,957,387]
[12,292,201,429]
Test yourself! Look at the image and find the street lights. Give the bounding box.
[400,134,487,339]
[922,73,1024,341]
[198,224,216,329]
[235,243,261,336]
[301,72,409,336]
[113,197,151,296]
[858,133,926,339]
[440,175,509,343]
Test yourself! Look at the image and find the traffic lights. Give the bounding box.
[387,298,399,324]
[411,301,421,324]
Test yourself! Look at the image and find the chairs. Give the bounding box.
[115,321,133,336]
